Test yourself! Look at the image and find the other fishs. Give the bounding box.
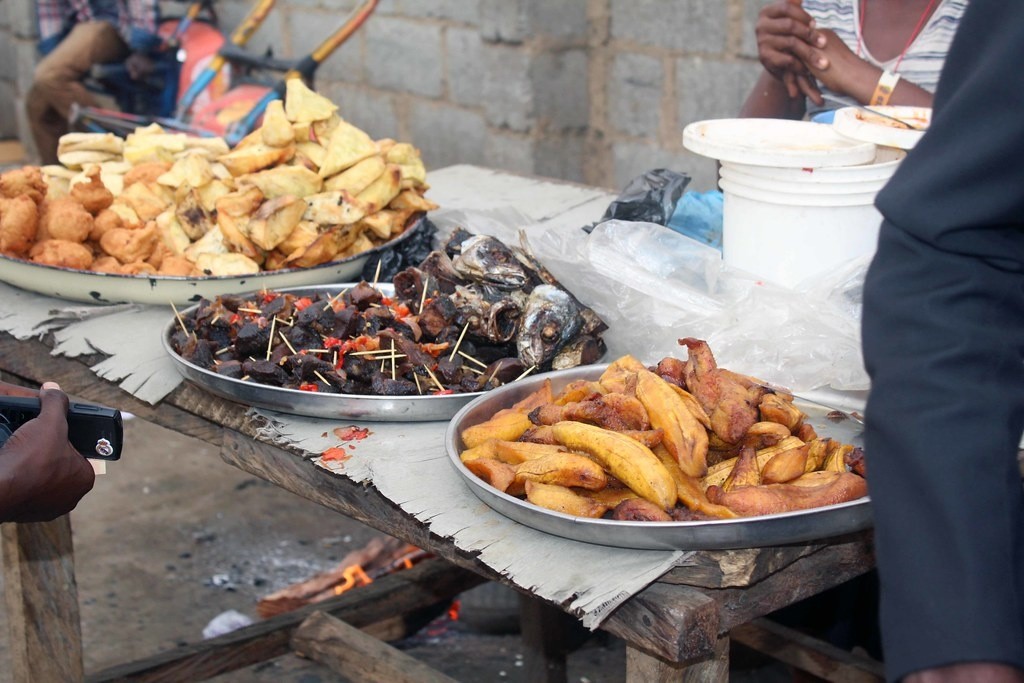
[392,227,611,370]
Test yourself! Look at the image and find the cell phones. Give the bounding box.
[0,396,123,462]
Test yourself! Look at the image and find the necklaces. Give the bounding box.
[856,0,934,72]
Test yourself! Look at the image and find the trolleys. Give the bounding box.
[68,0,377,153]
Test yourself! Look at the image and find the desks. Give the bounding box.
[0,164,883,683]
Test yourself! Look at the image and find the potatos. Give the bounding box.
[36,72,441,278]
[457,333,868,528]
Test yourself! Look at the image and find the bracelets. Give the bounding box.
[870,68,901,106]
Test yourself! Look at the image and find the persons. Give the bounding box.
[35,0,156,112]
[862,0,1024,683]
[669,0,969,250]
[0,382,95,524]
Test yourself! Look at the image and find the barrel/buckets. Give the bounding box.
[718,149,909,301]
